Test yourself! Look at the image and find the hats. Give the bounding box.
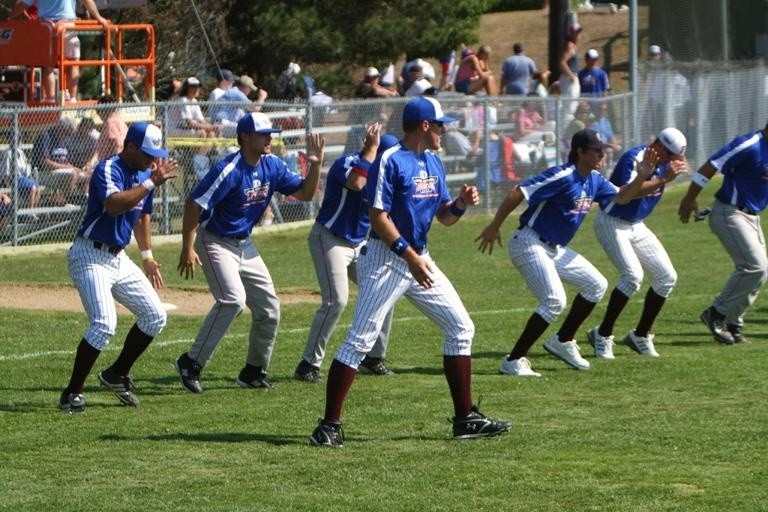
[236,112,282,134]
[584,49,599,60]
[460,48,474,56]
[567,23,583,37]
[217,69,238,81]
[572,127,615,150]
[240,75,257,91]
[364,67,381,79]
[648,45,661,55]
[185,76,202,88]
[380,134,400,151]
[125,121,170,158]
[659,127,687,156]
[403,96,457,123]
[408,65,422,73]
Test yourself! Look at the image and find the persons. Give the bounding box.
[650,55,696,136]
[678,121,768,347]
[5,0,109,102]
[590,102,623,166]
[500,42,540,95]
[517,90,556,148]
[57,120,179,414]
[646,44,663,61]
[471,90,504,188]
[341,67,396,154]
[176,112,327,393]
[209,69,239,117]
[292,119,402,384]
[586,126,690,360]
[579,49,612,98]
[95,96,124,162]
[404,64,436,99]
[0,190,14,233]
[444,110,483,161]
[563,102,590,153]
[454,44,503,107]
[1,133,41,222]
[558,22,584,115]
[306,95,512,447]
[65,116,96,168]
[31,117,92,196]
[473,127,661,377]
[167,76,223,137]
[438,35,455,91]
[400,48,436,87]
[209,75,269,124]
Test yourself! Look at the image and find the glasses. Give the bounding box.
[428,120,443,127]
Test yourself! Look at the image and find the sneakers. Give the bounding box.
[98,371,139,406]
[587,325,616,360]
[58,388,85,413]
[174,352,203,394]
[359,354,396,376]
[727,324,751,343]
[236,368,273,389]
[700,306,735,344]
[498,355,541,378]
[453,407,511,438]
[294,359,324,384]
[543,331,590,370]
[623,329,660,358]
[309,419,345,448]
